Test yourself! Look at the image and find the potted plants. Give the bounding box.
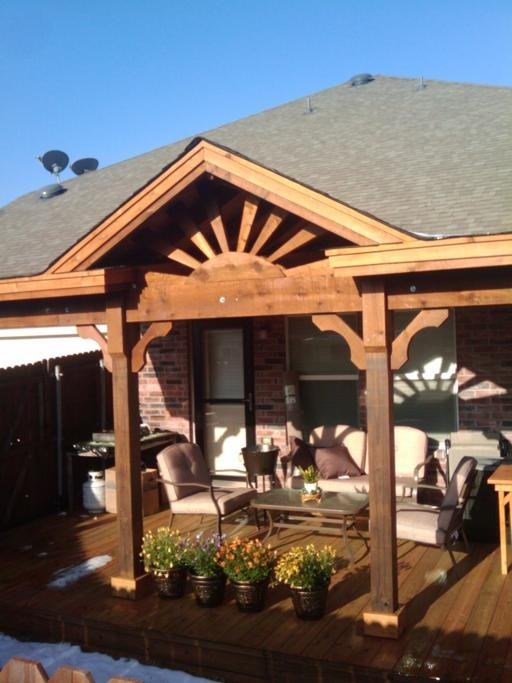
[297,463,323,496]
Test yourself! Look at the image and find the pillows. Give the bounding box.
[287,435,362,479]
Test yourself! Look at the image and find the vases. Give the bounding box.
[149,565,329,621]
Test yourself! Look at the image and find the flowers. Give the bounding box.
[139,525,347,584]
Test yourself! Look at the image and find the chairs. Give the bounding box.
[395,456,477,575]
[156,442,260,547]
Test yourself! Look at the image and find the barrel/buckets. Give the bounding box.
[241,446,279,476]
[459,457,502,545]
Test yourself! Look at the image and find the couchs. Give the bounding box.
[281,421,431,496]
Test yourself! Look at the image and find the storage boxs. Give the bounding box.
[105,464,159,516]
